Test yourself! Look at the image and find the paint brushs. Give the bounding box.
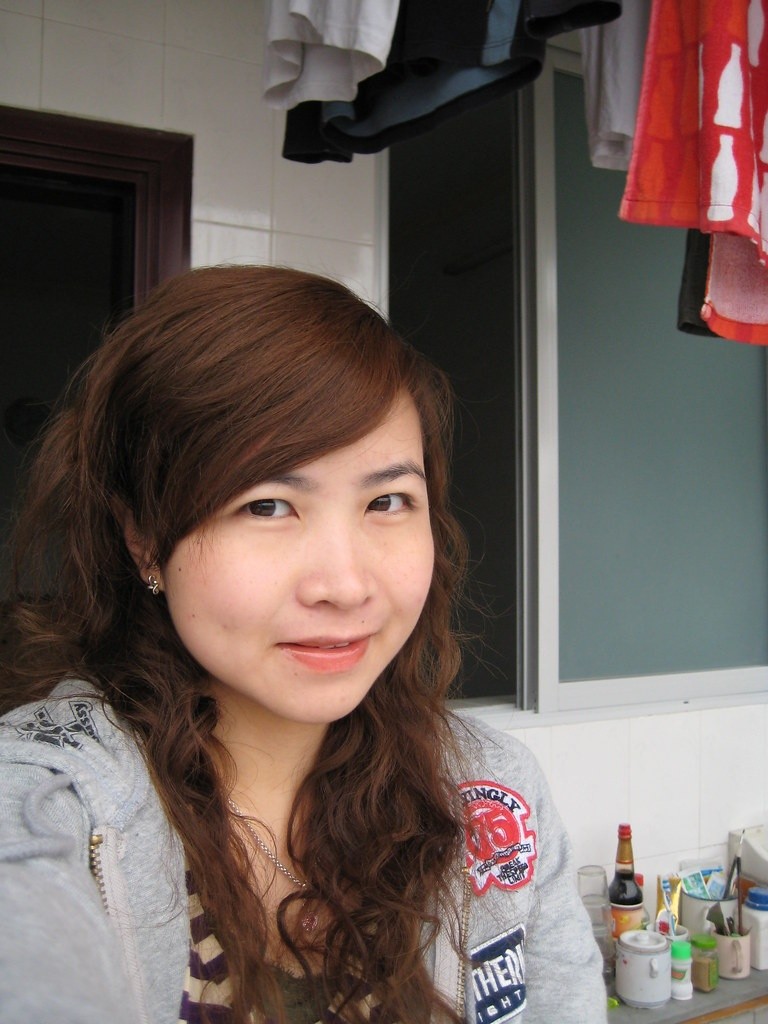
[734,843,746,935]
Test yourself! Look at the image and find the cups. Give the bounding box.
[707,925,751,980]
[681,887,739,935]
[615,930,672,1009]
[645,923,689,945]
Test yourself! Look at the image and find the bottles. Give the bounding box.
[690,933,720,992]
[577,865,615,985]
[632,873,649,931]
[741,887,768,971]
[609,824,644,979]
[669,941,694,1000]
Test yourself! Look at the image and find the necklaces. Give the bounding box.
[226,798,317,932]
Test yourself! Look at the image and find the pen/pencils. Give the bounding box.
[724,829,748,898]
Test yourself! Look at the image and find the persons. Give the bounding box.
[0,266,608,1024]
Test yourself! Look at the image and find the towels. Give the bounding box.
[618,0,768,345]
